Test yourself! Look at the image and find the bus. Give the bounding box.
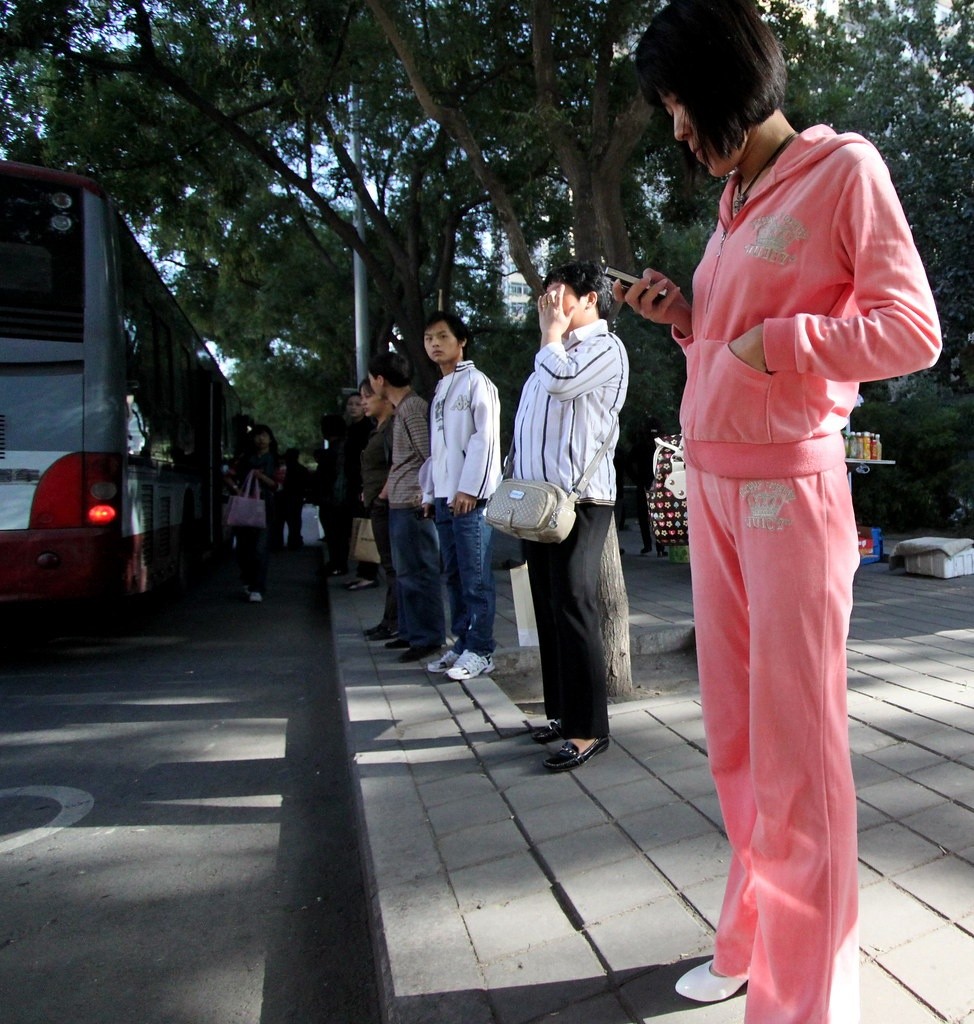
[1,158,240,613]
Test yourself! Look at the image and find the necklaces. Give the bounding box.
[732,130,799,214]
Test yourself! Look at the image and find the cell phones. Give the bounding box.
[605,267,668,299]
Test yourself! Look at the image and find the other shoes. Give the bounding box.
[362,623,396,640]
[342,576,381,593]
[242,585,263,602]
[385,640,442,664]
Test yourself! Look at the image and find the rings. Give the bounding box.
[541,300,555,307]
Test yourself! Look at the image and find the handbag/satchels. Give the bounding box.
[220,468,267,528]
[347,517,383,564]
[646,434,689,546]
[481,478,576,544]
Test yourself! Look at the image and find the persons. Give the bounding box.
[359,378,400,641]
[219,409,352,605]
[513,255,629,773]
[614,444,631,555]
[340,391,382,593]
[417,311,503,682]
[612,1,941,1023]
[368,351,447,663]
[629,416,668,557]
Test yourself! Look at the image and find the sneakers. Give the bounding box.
[426,649,494,680]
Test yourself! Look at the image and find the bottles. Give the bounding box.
[842,429,881,460]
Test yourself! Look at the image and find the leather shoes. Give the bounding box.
[675,959,747,1004]
[532,720,609,770]
